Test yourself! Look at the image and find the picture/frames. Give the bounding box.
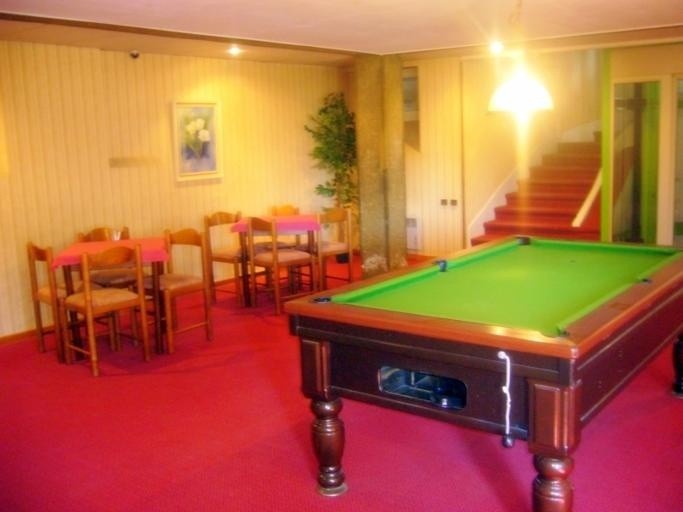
[173,99,225,182]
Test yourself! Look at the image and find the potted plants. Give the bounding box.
[304,90,359,262]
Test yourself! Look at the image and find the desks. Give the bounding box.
[52,237,170,360]
[282,233,683,512]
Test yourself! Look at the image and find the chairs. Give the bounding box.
[27,241,117,364]
[64,243,152,377]
[129,203,356,354]
[77,226,146,351]
